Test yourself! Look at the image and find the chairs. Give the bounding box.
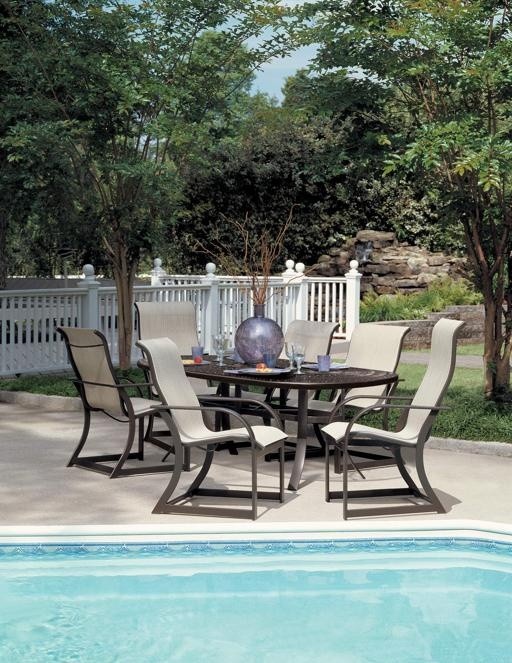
[220,318,341,462]
[134,300,240,455]
[320,316,467,520]
[134,334,287,521]
[276,322,412,474]
[55,325,192,479]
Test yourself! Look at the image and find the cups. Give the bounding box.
[192,346,204,362]
[317,355,331,373]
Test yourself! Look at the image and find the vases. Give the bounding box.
[235,305,285,367]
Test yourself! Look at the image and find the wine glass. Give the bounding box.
[285,342,306,375]
[211,335,227,367]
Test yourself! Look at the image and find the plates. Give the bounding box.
[223,366,291,377]
[301,363,351,370]
[182,359,210,366]
